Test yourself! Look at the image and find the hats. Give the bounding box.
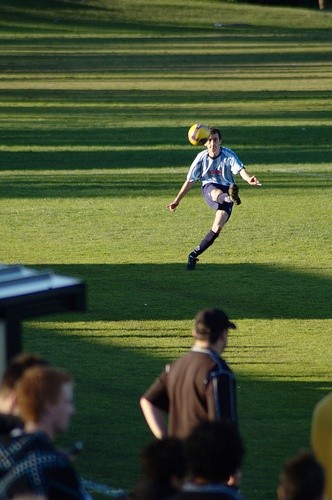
[196,309,236,335]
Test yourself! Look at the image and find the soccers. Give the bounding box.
[187,124,210,146]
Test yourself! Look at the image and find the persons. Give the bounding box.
[127,421,325,500]
[138,308,241,488]
[0,354,49,443]
[0,365,82,500]
[166,128,262,271]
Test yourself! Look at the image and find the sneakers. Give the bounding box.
[187,252,199,270]
[229,183,241,205]
